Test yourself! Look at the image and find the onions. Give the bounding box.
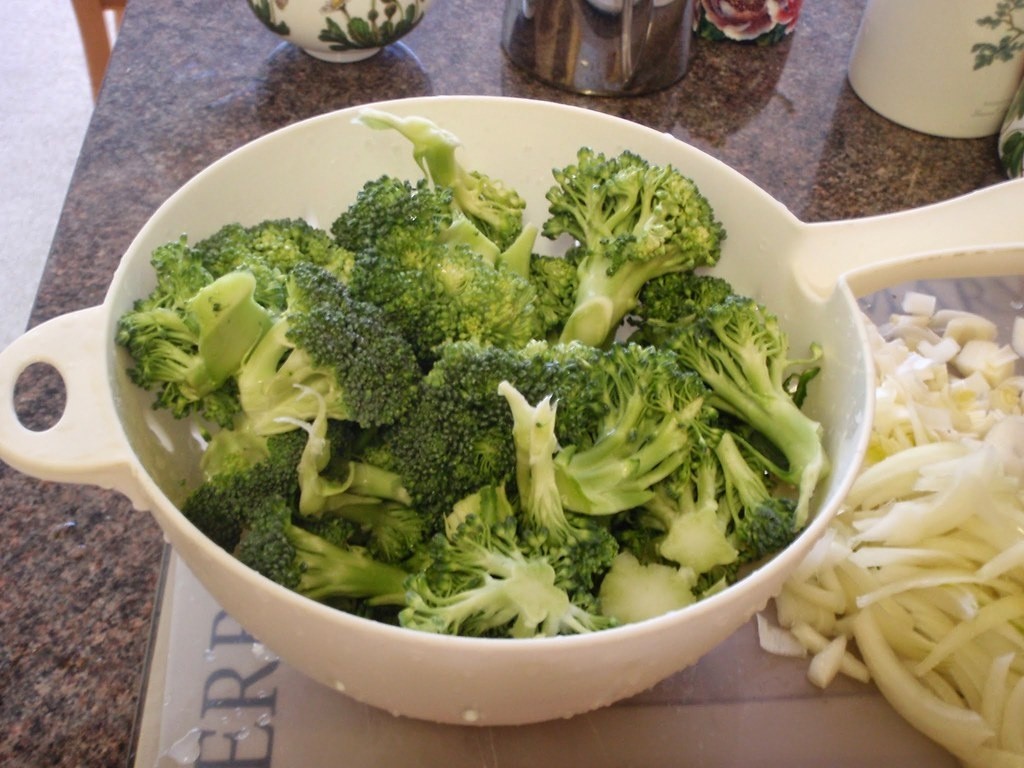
[757,336,1024,768]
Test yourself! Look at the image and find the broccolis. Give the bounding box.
[113,109,833,640]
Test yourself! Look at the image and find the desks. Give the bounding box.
[0,0,1024,767]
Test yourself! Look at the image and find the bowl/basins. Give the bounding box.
[0,95,1024,726]
[246,0,433,64]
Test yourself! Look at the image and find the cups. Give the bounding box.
[500,0,696,97]
[691,0,804,46]
[847,0,1024,139]
